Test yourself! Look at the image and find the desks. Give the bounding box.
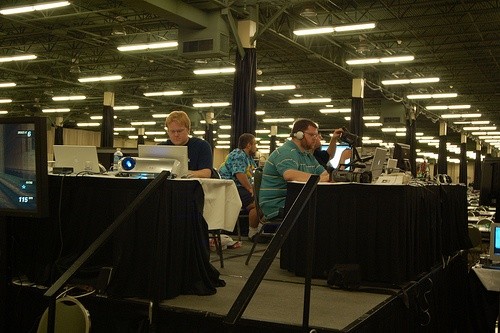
[22,174,242,302]
[279,181,500,293]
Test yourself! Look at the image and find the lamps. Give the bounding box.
[0,0,500,162]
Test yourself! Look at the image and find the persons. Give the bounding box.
[259,119,329,217]
[311,128,351,171]
[157,111,213,178]
[219,133,263,239]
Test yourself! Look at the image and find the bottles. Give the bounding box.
[114,148,123,173]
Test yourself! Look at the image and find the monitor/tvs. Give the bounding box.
[320,145,352,171]
[490,223,500,262]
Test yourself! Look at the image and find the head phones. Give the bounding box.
[290,131,304,140]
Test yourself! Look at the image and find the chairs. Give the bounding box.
[209,168,283,267]
[37,296,89,333]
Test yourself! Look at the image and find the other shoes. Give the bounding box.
[247,226,275,243]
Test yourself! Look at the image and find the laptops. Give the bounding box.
[138,145,188,178]
[53,145,99,173]
[371,148,387,180]
